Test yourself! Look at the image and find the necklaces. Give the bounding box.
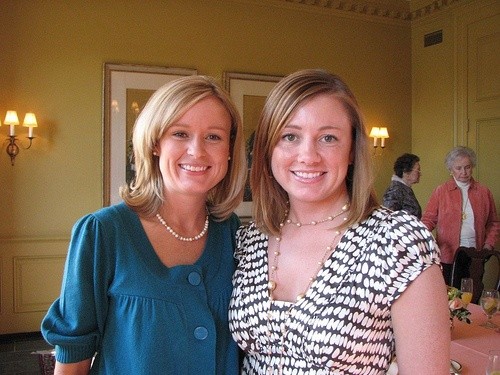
[285,201,352,226]
[155,205,210,241]
[462,197,468,219]
[267,216,348,314]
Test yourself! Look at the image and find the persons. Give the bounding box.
[228,69,453,375]
[40,75,248,375]
[382,152,422,221]
[420,146,500,304]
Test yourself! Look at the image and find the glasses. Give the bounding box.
[410,167,422,173]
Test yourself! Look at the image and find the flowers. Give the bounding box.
[447,286,472,325]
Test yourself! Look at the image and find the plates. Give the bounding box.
[449,359,463,373]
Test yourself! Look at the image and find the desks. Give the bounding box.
[446,302,500,375]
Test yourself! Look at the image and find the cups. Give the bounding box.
[485,348,500,375]
[461,278,473,307]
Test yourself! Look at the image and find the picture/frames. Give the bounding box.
[220,70,285,218]
[101,61,198,208]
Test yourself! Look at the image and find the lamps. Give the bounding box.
[0,110,38,166]
[369,126,390,149]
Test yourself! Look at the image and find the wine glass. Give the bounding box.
[479,289,499,328]
